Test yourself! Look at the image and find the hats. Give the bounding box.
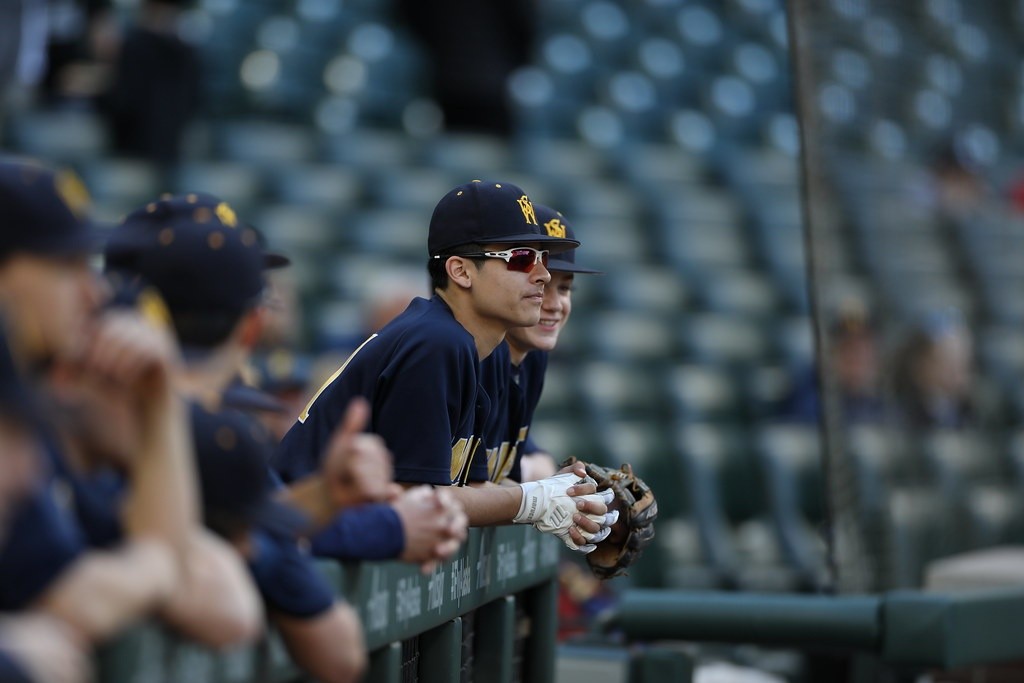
[426,180,581,256]
[529,203,603,273]
[0,162,156,260]
[111,191,292,349]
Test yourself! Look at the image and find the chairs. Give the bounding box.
[14,107,1024,680]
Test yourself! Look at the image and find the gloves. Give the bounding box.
[511,471,619,555]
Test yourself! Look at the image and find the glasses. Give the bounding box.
[460,247,550,273]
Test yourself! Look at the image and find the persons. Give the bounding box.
[472,196,605,512]
[106,189,402,682]
[240,448,470,577]
[276,180,623,556]
[23,4,1021,433]
[0,403,103,682]
[3,155,269,647]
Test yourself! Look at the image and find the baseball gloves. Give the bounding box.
[568,465,659,580]
